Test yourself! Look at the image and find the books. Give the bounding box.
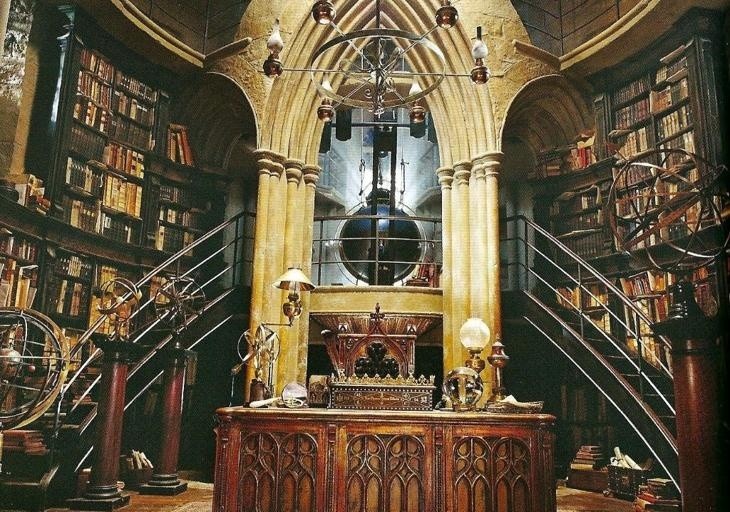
[126,344,199,434]
[62,123,198,258]
[559,384,682,511]
[73,49,198,168]
[555,265,711,373]
[546,56,706,266]
[2,228,174,372]
[2,374,98,456]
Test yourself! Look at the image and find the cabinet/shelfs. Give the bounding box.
[2,14,216,379]
[550,9,730,391]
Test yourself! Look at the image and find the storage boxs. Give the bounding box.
[608,464,658,503]
[566,463,609,492]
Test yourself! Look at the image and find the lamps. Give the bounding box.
[260,0,493,127]
[244,263,315,408]
[453,317,490,375]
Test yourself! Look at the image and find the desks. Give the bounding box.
[211,397,562,512]
